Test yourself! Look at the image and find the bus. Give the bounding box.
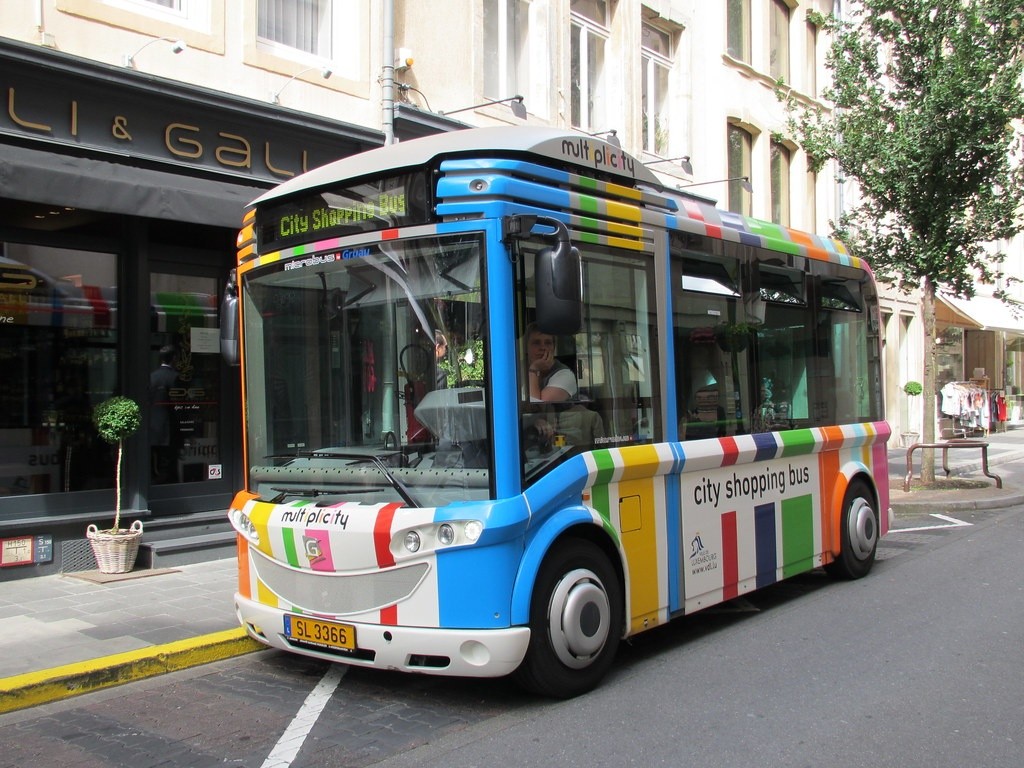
[220,121,900,699]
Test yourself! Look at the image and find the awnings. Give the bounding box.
[934,285,1024,334]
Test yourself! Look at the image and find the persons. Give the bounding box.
[521,320,579,440]
[558,395,605,448]
[435,328,449,389]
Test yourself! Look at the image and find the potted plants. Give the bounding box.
[900,381,923,448]
[86,397,143,573]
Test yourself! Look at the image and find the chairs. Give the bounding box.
[555,358,793,439]
